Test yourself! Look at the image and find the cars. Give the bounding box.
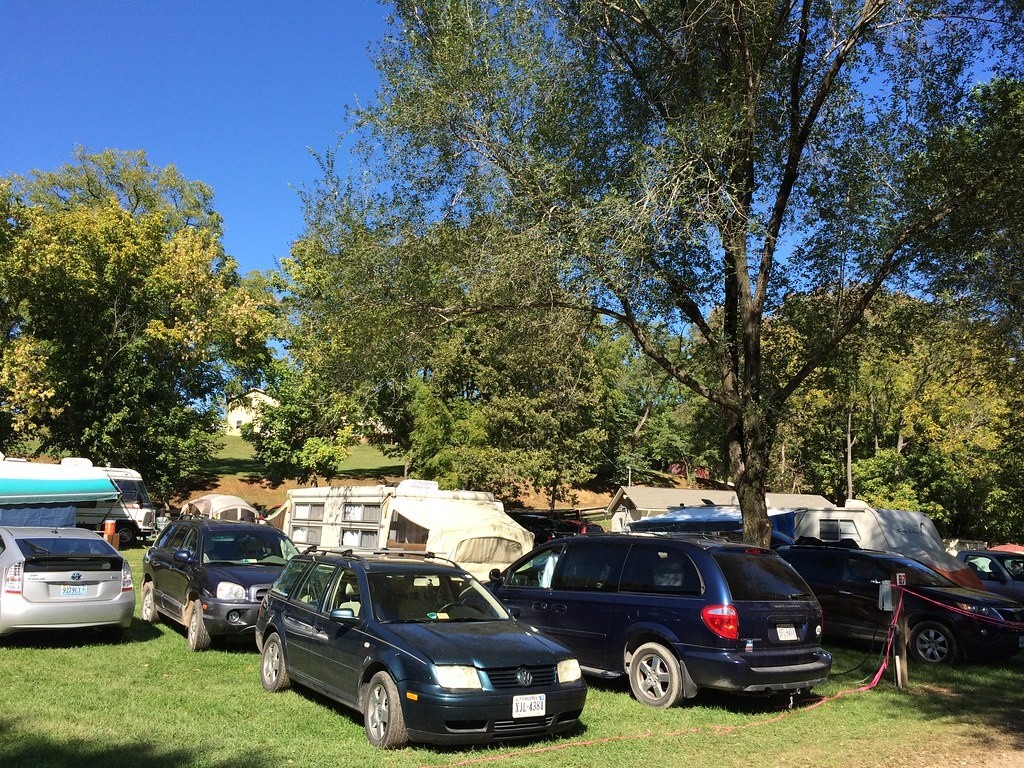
[512,513,605,547]
[955,548,1024,603]
[0,525,136,642]
[769,542,1024,670]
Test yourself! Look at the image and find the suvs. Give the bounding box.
[474,531,833,713]
[141,512,322,653]
[254,543,588,751]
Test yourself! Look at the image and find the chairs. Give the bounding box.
[203,538,218,559]
[339,578,386,622]
[154,522,168,538]
[989,561,1005,580]
[397,577,432,620]
[967,562,983,580]
[240,539,264,561]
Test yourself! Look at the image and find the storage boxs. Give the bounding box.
[105,520,116,535]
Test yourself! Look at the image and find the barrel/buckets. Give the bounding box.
[104,520,115,534]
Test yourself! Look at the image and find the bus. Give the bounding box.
[75,462,156,547]
